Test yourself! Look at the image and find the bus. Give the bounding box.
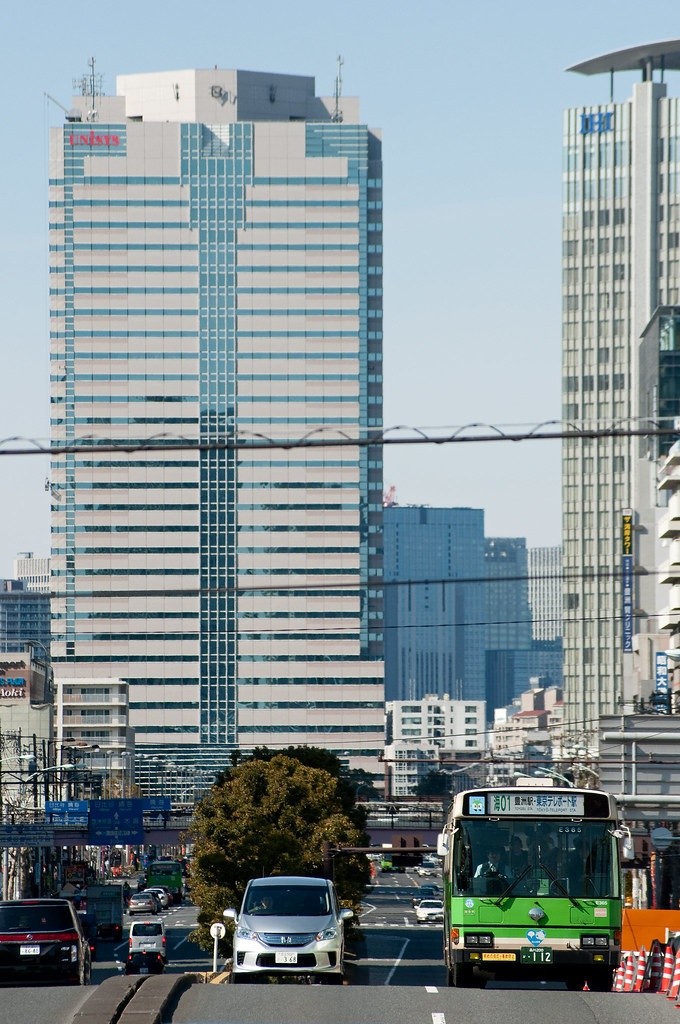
[437,776,635,992]
[144,861,183,904]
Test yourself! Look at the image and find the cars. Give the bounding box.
[417,862,437,879]
[410,887,441,909]
[125,951,165,975]
[138,891,164,913]
[136,877,147,893]
[142,887,169,911]
[414,899,445,924]
[153,886,174,907]
[421,883,441,893]
[222,876,354,986]
[128,893,157,915]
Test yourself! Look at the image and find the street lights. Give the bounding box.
[78,748,222,800]
[47,736,76,794]
[2,764,75,901]
[73,744,101,799]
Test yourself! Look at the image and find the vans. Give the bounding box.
[126,921,170,960]
[0,898,93,986]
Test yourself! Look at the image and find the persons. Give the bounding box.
[123,881,131,905]
[511,836,528,867]
[568,837,581,863]
[474,849,512,878]
[248,897,274,913]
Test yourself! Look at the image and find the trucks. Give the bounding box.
[86,884,124,942]
